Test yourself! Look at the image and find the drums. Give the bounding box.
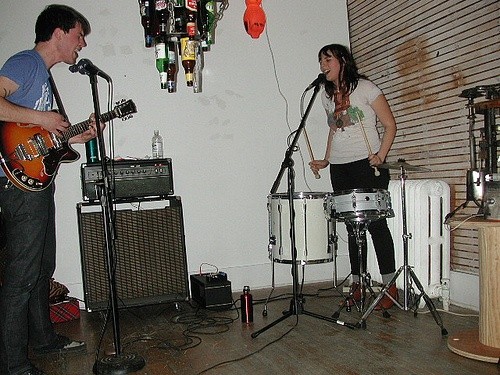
[270,190,336,265]
[325,185,392,222]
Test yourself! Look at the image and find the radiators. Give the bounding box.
[344,180,450,312]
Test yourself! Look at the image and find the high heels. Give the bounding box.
[375,283,399,311]
[338,284,363,307]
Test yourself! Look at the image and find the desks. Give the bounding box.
[447,216,500,363]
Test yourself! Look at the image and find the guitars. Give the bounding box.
[1,99,138,191]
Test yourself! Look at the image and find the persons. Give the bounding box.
[0,3,106,374]
[309,44,398,311]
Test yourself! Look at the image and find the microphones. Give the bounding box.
[307,74,325,90]
[79,59,110,81]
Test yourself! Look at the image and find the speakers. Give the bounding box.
[78,196,190,313]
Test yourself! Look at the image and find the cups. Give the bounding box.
[85,137,99,163]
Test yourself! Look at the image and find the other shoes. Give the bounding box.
[22,368,48,375]
[34,334,87,355]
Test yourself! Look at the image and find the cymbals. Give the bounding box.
[375,159,432,175]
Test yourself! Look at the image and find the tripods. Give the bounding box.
[250,85,450,339]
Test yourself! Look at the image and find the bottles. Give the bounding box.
[152,129,163,159]
[138,0,168,48]
[170,0,215,54]
[181,36,198,85]
[193,43,201,92]
[167,40,176,93]
[155,35,169,89]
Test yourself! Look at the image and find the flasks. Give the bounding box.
[241,285,253,323]
